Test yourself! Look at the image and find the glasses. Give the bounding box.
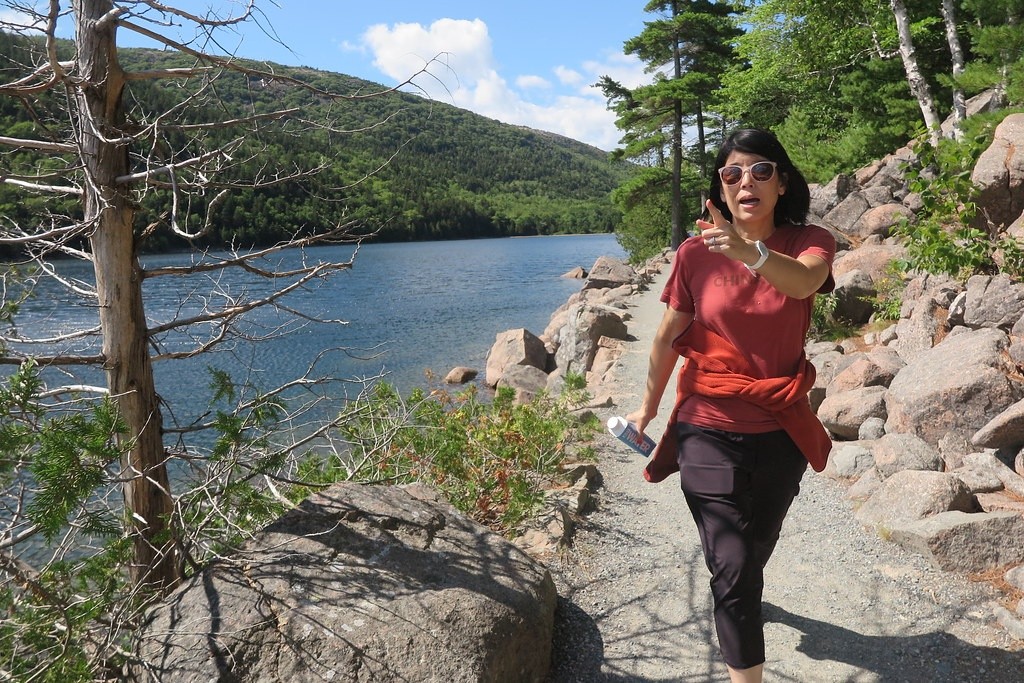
[717,161,776,187]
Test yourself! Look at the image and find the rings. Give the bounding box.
[713,236,715,245]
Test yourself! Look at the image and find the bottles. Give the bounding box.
[607,415,657,458]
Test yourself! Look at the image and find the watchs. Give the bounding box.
[743,240,768,278]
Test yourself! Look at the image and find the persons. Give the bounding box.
[624,128,834,683]
